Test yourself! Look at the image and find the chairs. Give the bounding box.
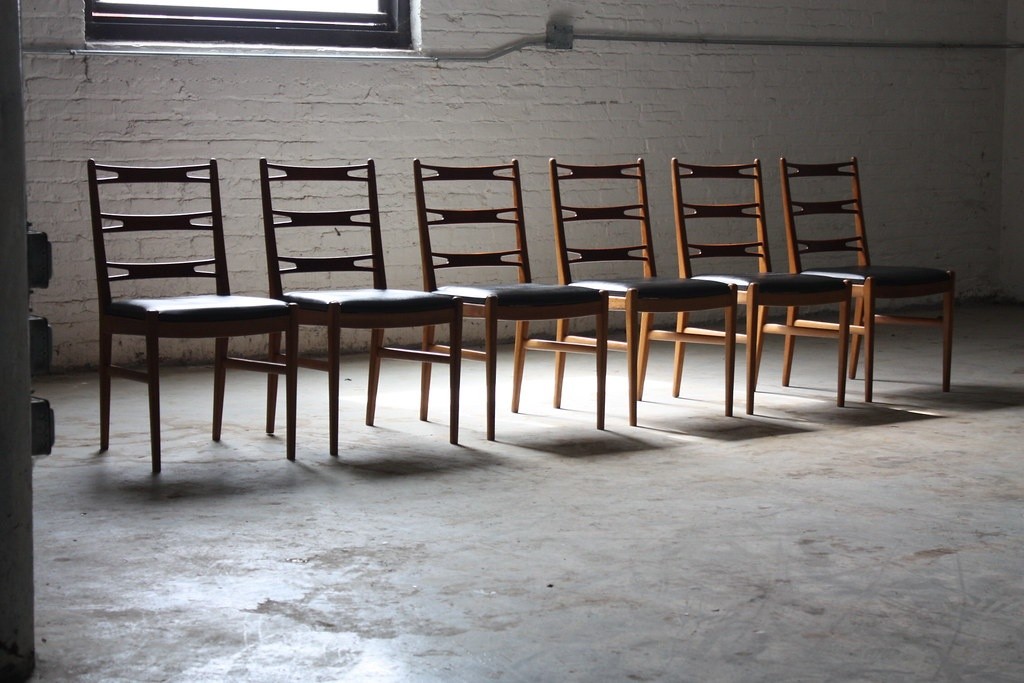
[413,157,610,441]
[88,154,301,472]
[549,157,738,426]
[670,157,852,415]
[261,156,462,455]
[778,157,956,402]
